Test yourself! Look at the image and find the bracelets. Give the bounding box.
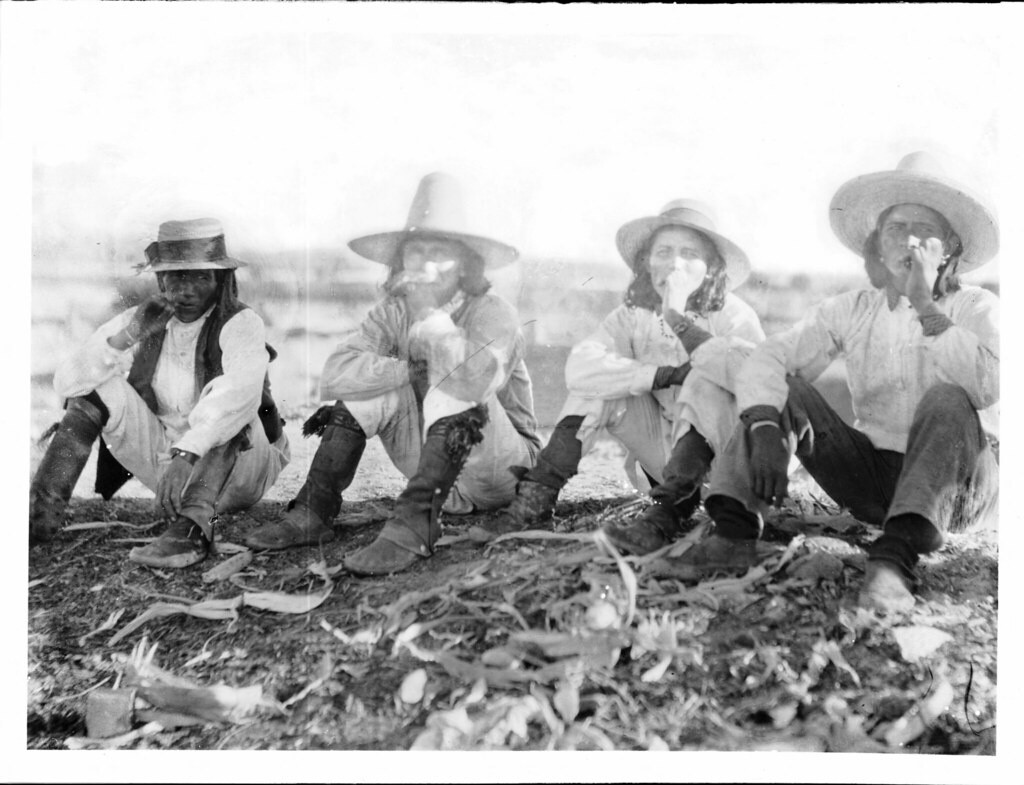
[656,365,675,388]
[750,421,779,431]
[125,329,138,344]
[673,318,692,335]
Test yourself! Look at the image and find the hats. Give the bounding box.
[615,197,753,291]
[829,150,999,274]
[347,172,520,269]
[135,218,249,273]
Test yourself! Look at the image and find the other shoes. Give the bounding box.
[650,525,756,580]
[342,518,441,575]
[129,522,210,568]
[247,506,335,549]
[30,505,61,537]
[858,560,915,615]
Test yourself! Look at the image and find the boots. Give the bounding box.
[466,479,556,545]
[596,493,676,556]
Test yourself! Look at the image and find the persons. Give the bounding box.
[647,152,1001,618]
[30,217,289,566]
[465,202,764,558]
[253,172,543,574]
